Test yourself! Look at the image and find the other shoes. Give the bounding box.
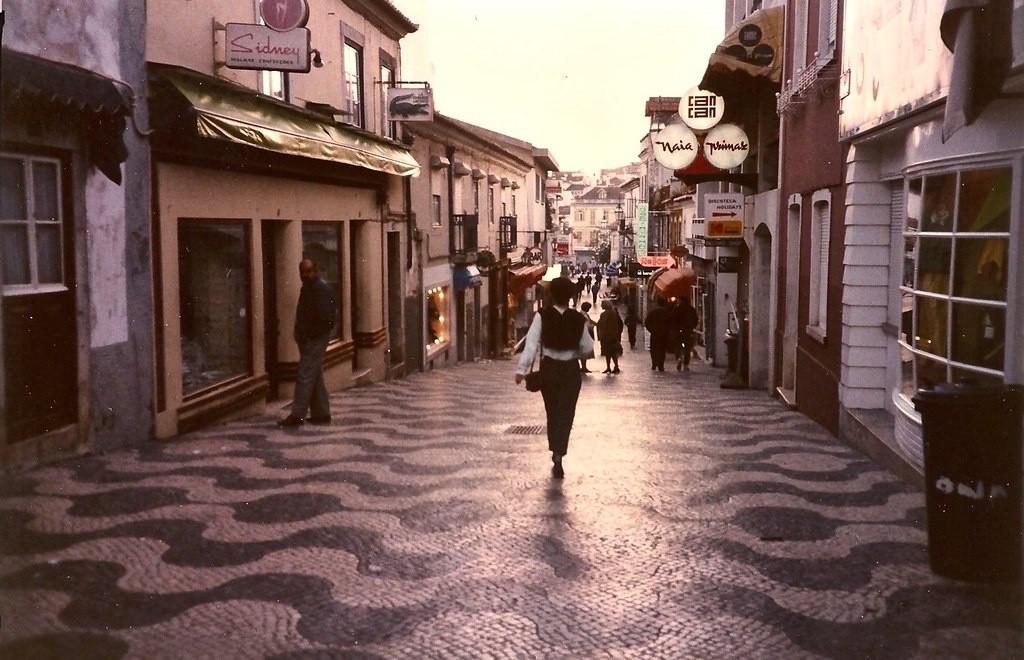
[305,416,330,422]
[676,358,682,370]
[582,368,591,372]
[277,416,304,426]
[684,365,689,369]
[552,457,564,475]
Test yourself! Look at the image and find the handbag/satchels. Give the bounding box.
[525,371,544,392]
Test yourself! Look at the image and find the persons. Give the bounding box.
[597,301,621,374]
[276,259,337,426]
[592,282,599,303]
[578,303,596,372]
[645,295,698,372]
[596,272,602,285]
[601,307,623,356]
[569,261,592,307]
[515,277,593,476]
[624,311,638,348]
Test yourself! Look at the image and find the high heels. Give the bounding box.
[611,368,620,373]
[602,368,611,373]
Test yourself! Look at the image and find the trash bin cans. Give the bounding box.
[912,374,1024,583]
[724,332,749,382]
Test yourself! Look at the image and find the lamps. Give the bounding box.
[312,49,324,68]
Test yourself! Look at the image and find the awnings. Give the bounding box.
[698,6,784,96]
[454,264,483,292]
[606,260,624,276]
[148,62,420,178]
[653,267,695,294]
[508,264,547,290]
[0,48,134,117]
